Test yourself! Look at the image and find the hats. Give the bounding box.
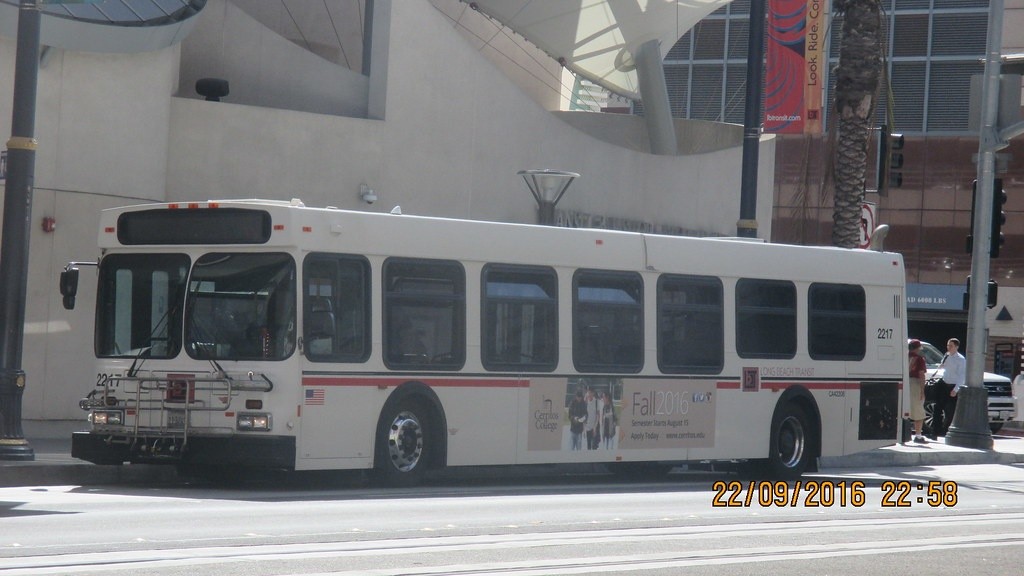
[909,340,921,350]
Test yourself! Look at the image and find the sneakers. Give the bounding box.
[914,437,929,444]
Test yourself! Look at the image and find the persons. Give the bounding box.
[930,338,968,442]
[908,340,929,444]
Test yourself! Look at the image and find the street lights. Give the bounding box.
[518,169,579,226]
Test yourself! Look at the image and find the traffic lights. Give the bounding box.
[886,133,904,189]
[990,178,1010,259]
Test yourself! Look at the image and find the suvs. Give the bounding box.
[908,339,1017,434]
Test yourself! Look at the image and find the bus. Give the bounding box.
[59,199,909,483]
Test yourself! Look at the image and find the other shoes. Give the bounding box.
[925,433,937,441]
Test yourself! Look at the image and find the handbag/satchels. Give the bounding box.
[924,379,938,402]
